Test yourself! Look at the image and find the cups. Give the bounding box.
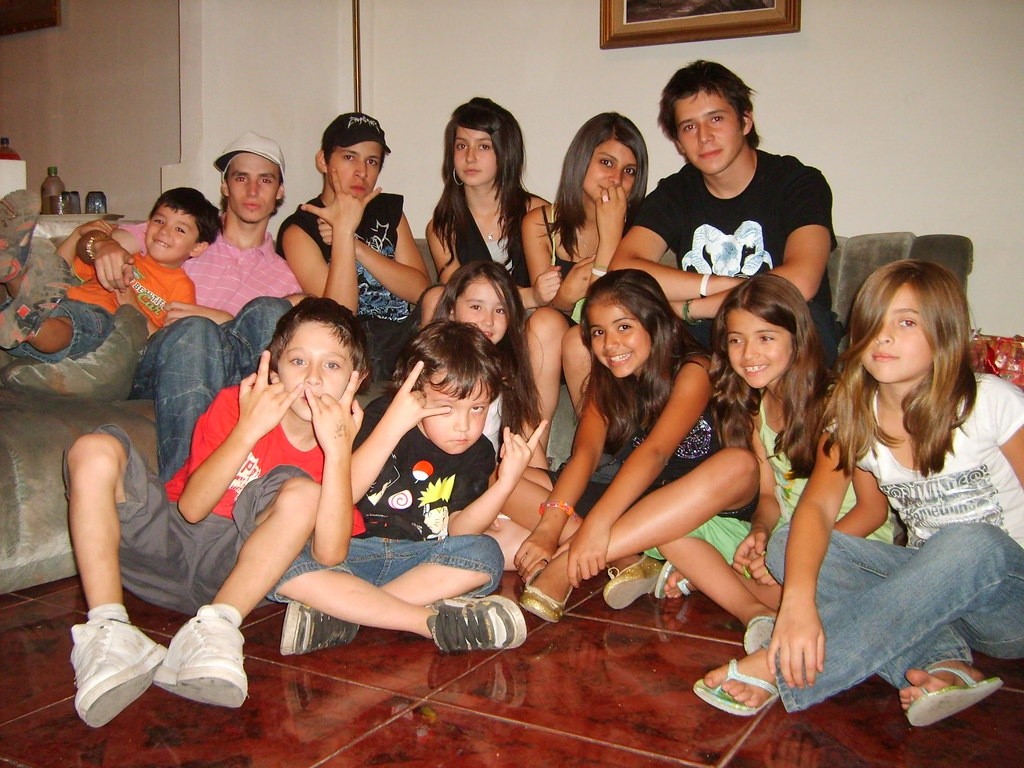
[49,194,71,215]
[60,191,81,214]
[86,191,107,214]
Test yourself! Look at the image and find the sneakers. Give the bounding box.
[152,605,248,707]
[0,190,41,281]
[280,601,360,655]
[427,597,527,653]
[71,618,168,727]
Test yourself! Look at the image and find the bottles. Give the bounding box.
[0,137,21,160]
[40,167,65,214]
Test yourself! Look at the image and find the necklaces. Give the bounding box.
[467,207,500,240]
[576,227,598,258]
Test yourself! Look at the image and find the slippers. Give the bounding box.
[904,667,1003,726]
[744,616,778,654]
[694,659,780,716]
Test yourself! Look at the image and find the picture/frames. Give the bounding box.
[599,0,804,49]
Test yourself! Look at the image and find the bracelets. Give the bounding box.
[682,299,705,326]
[593,262,608,269]
[592,268,607,277]
[699,273,711,299]
[539,501,578,522]
[85,234,108,260]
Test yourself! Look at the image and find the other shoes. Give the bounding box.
[518,569,574,624]
[604,554,663,609]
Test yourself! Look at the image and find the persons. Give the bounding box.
[263,261,894,656]
[0,59,846,484]
[61,297,370,728]
[693,258,1024,727]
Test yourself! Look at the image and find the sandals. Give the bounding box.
[655,561,692,599]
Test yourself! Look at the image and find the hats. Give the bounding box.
[322,113,391,153]
[214,129,285,182]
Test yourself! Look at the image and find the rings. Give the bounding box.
[602,196,608,202]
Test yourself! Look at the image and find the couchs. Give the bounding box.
[0,210,973,597]
